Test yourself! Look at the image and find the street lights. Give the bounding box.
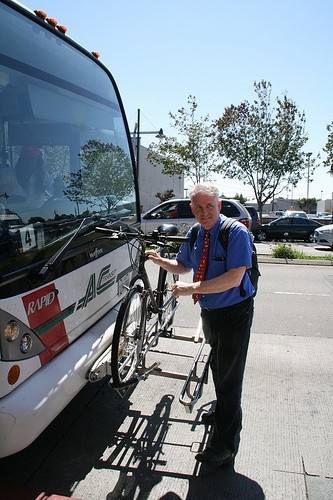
[130,122,166,163]
[305,152,313,214]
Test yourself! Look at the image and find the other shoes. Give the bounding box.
[195,442,234,464]
[202,411,214,424]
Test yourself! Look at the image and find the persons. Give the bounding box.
[13,143,55,211]
[162,205,177,218]
[144,183,261,465]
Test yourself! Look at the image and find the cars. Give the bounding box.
[314,223,333,251]
[306,211,333,225]
[261,216,325,243]
[257,209,308,225]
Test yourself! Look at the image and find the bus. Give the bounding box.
[0,0,151,459]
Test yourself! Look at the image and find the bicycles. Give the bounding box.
[92,223,191,388]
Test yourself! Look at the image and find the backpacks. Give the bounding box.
[191,218,261,297]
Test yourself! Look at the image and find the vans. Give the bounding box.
[140,198,252,237]
[244,206,262,243]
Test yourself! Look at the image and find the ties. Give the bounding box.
[192,231,209,305]
